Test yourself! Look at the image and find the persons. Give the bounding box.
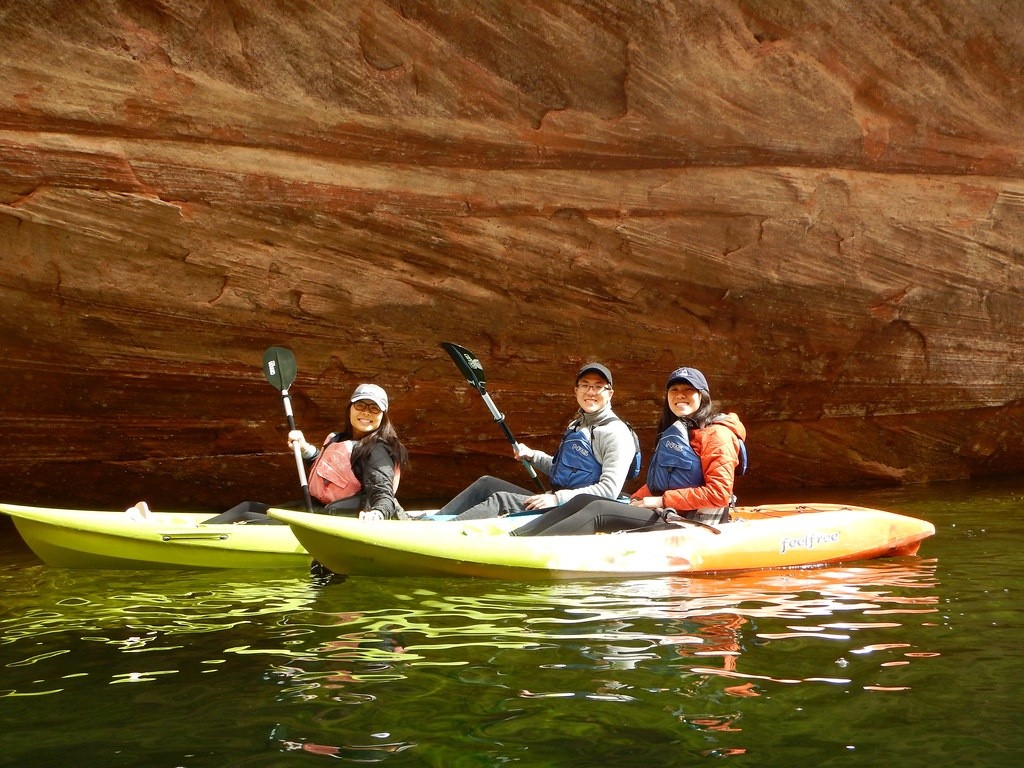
[394,362,635,521]
[125,383,409,524]
[464,368,746,537]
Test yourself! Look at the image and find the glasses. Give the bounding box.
[575,383,613,395]
[354,400,381,414]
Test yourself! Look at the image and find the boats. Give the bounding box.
[0,503,440,573]
[268,502,935,586]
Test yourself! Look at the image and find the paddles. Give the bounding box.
[648,504,721,536]
[438,338,548,494]
[263,347,341,582]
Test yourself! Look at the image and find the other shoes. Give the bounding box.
[124,507,144,523]
[393,498,421,521]
[135,501,154,521]
[462,525,509,538]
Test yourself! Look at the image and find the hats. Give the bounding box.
[350,384,388,413]
[576,363,613,387]
[665,367,709,394]
